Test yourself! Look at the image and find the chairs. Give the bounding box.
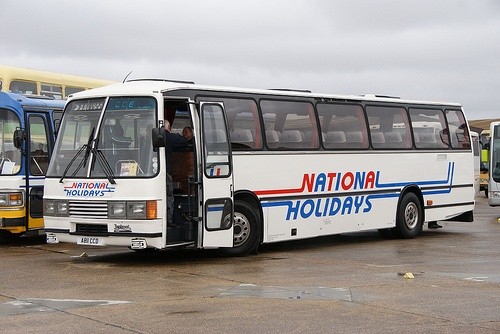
[202,128,463,150]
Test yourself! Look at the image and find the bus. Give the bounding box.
[41,78,476,258]
[367,121,481,207]
[0,63,122,238]
